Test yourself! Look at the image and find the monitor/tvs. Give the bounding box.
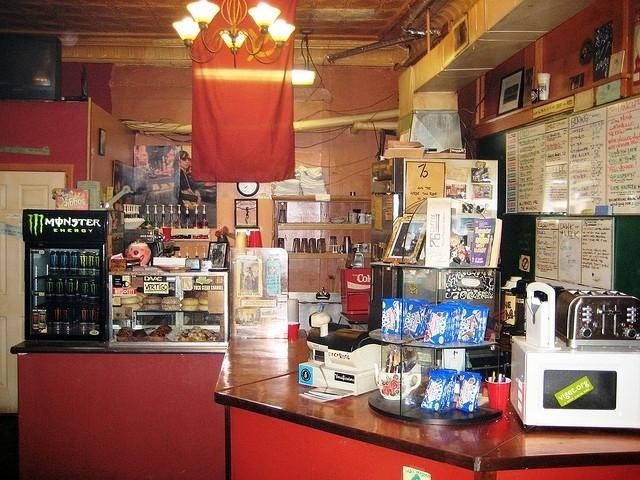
[0,32,62,100]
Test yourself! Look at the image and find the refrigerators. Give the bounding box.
[22,208,125,347]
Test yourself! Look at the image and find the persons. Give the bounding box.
[185,159,194,175]
[171,150,201,210]
[398,224,417,255]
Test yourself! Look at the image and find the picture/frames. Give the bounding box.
[497,67,524,114]
[234,199,258,229]
[207,242,228,269]
[386,212,425,263]
[99,127,107,154]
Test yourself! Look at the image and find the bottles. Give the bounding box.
[144,203,209,239]
[330,235,337,251]
[278,209,286,223]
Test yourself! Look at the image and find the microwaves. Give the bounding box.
[509,336,639,429]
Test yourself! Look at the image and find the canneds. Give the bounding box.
[366,214,372,224]
[31,250,99,330]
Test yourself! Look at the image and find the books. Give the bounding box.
[462,216,503,267]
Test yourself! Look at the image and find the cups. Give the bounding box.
[485,378,512,411]
[235,231,262,248]
[288,323,300,342]
[278,238,284,248]
[293,238,326,254]
[349,213,372,224]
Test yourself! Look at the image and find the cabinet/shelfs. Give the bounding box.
[369,262,503,425]
[108,271,226,345]
[272,196,374,260]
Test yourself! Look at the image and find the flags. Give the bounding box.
[183,1,301,186]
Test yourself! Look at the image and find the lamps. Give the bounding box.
[171,0,296,69]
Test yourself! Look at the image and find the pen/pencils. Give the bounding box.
[487,371,506,382]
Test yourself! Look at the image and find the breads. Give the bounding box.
[109,240,219,343]
